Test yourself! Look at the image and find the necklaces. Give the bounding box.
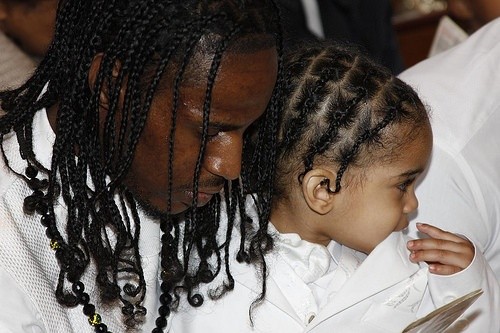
[14,107,176,333]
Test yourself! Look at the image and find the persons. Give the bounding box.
[0,0,306,333]
[2,0,500,272]
[240,35,500,333]
